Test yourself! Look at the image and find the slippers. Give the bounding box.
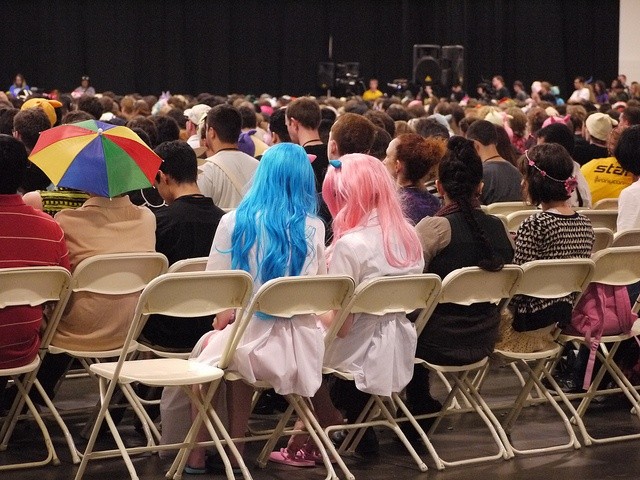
[184,465,206,474]
[269,444,337,466]
[208,455,247,474]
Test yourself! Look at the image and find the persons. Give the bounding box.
[583,111,609,160]
[613,122,639,412]
[580,155,636,204]
[466,119,527,203]
[1,130,71,411]
[157,139,327,473]
[534,121,591,208]
[264,154,417,467]
[197,102,260,208]
[513,142,596,351]
[27,193,159,411]
[97,138,227,441]
[549,73,639,126]
[337,135,514,457]
[381,135,441,223]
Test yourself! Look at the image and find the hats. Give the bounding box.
[542,114,571,129]
[238,130,256,156]
[183,104,213,125]
[586,113,618,147]
[484,107,514,127]
[20,98,63,127]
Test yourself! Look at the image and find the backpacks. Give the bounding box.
[564,285,639,390]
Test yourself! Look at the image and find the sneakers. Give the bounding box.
[331,427,379,453]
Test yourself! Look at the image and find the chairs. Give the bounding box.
[163,255,211,273]
[488,200,529,214]
[611,231,639,246]
[561,246,639,450]
[306,273,444,480]
[79,270,255,479]
[587,222,613,247]
[196,274,358,480]
[5,252,169,460]
[394,265,509,470]
[0,265,74,474]
[578,208,622,227]
[510,257,596,459]
[509,210,543,229]
